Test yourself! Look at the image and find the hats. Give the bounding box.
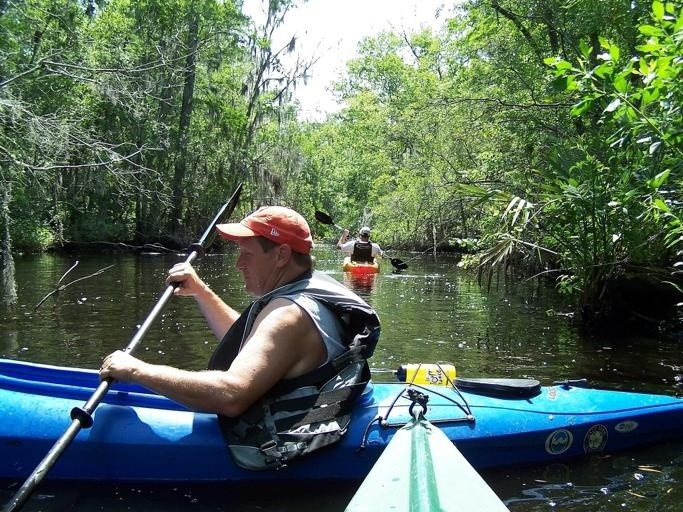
[358,227,370,236]
[215,206,313,255]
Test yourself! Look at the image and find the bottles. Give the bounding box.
[391,363,457,386]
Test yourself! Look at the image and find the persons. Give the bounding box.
[98,206,381,473]
[336,226,383,272]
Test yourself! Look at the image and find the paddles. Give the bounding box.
[4,182,241,512]
[314,210,409,271]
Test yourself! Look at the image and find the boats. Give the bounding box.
[343,259,380,274]
